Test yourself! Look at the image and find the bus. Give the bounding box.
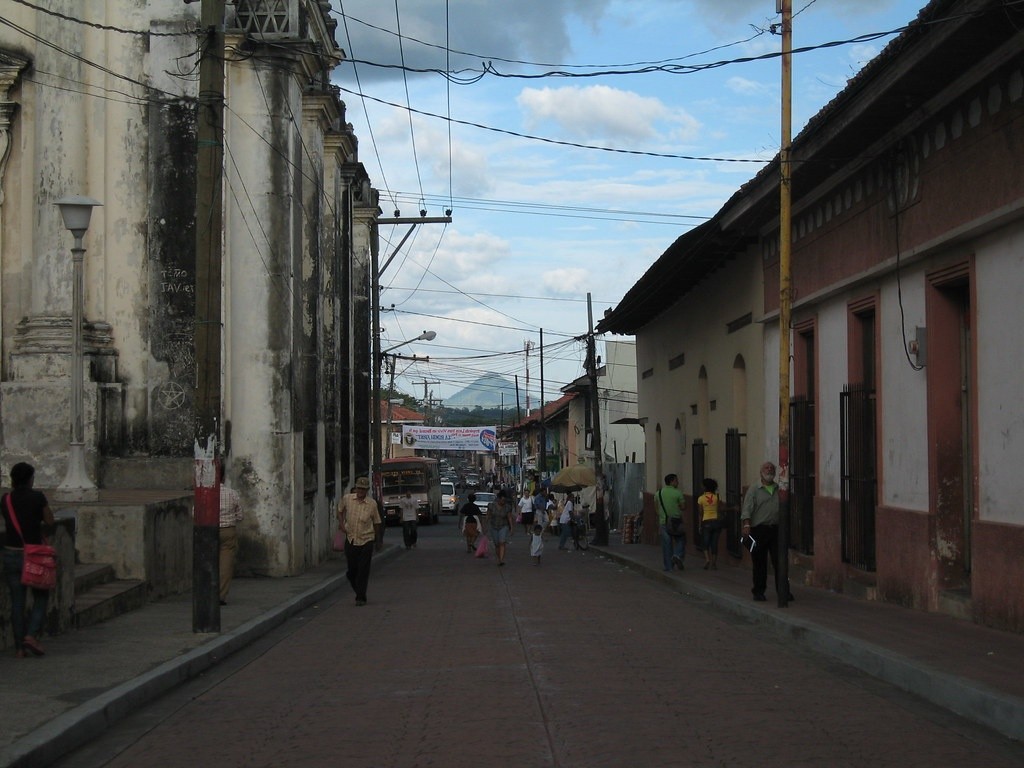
[378,456,441,524]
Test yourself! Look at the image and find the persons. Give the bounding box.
[528,525,549,567]
[654,474,688,572]
[461,467,523,502]
[516,486,581,537]
[191,466,245,606]
[697,478,739,571]
[399,490,421,551]
[0,462,57,659]
[741,461,794,602]
[559,494,580,550]
[336,477,384,606]
[457,493,483,554]
[484,493,514,567]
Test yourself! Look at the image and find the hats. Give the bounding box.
[356,477,370,489]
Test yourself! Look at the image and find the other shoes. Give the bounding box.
[753,594,767,602]
[673,555,684,570]
[355,599,366,607]
[703,560,710,570]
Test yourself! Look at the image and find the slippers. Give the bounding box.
[14,649,26,659]
[22,635,45,656]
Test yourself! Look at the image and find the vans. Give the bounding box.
[439,482,458,514]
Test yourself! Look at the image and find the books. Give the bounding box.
[741,534,756,552]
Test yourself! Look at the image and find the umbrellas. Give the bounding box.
[549,464,595,516]
[542,476,588,499]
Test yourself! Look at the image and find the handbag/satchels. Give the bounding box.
[476,535,490,558]
[20,544,56,590]
[665,516,687,536]
[332,530,346,552]
[515,515,522,523]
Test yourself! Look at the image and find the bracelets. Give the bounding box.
[743,525,751,527]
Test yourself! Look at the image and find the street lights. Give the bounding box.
[58,193,111,504]
[374,330,438,549]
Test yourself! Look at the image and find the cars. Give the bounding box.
[440,459,482,488]
[474,493,497,515]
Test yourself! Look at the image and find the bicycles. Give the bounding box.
[572,520,589,550]
